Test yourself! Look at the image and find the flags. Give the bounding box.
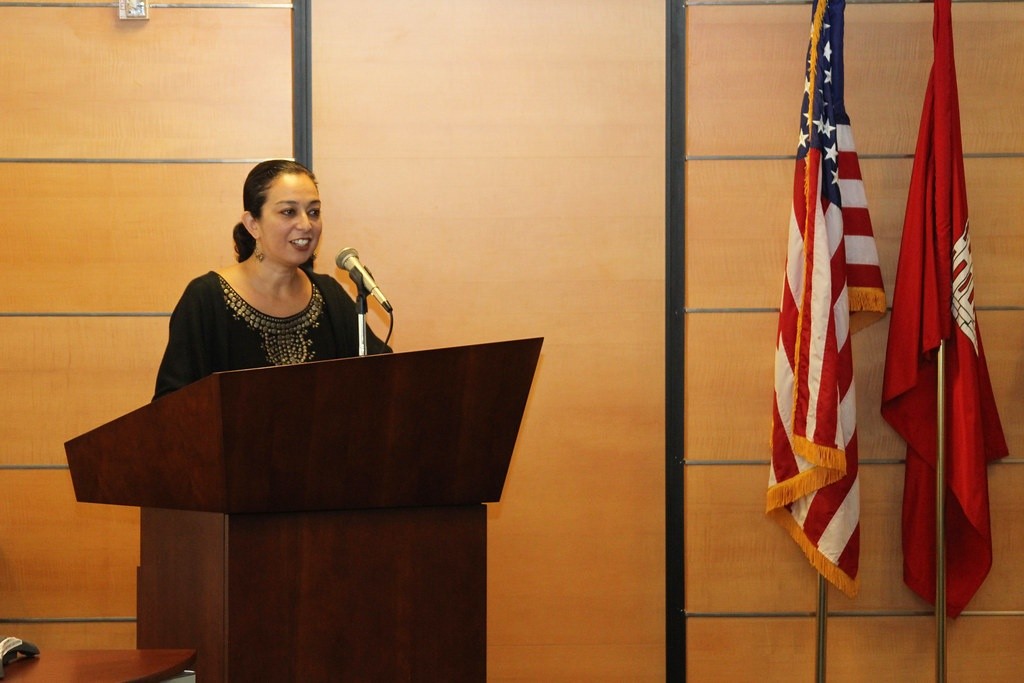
[877,0,1009,620]
[763,0,888,600]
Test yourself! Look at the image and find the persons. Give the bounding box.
[152,160,394,400]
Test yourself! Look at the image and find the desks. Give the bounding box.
[0,649,197,683]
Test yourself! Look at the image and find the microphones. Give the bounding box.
[336,247,392,315]
